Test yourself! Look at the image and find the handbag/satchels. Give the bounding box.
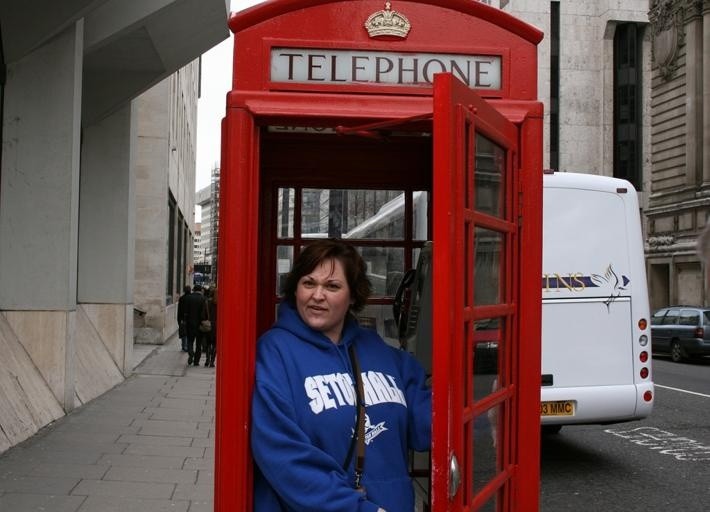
[200,320,212,332]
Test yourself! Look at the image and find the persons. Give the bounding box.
[178,281,217,367]
[249,238,500,511]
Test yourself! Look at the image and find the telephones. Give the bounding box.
[392,240,434,378]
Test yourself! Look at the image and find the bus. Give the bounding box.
[340,167,656,442]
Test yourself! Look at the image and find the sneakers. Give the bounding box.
[205,362,215,367]
[188,352,193,365]
[194,362,199,366]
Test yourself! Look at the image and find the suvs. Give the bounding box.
[649,303,709,365]
